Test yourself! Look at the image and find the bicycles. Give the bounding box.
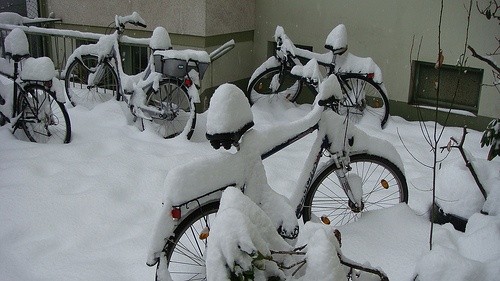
[146,95,408,281]
[65,20,210,140]
[247,35,389,126]
[0,52,71,144]
